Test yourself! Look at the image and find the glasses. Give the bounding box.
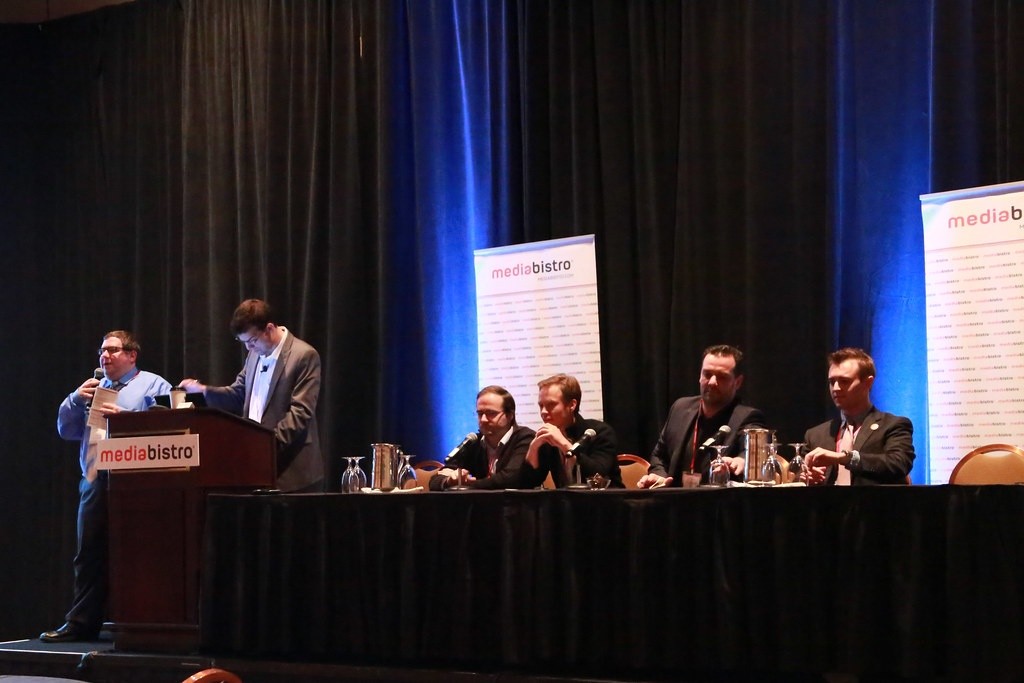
[97,347,133,356]
[234,330,266,344]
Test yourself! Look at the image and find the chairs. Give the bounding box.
[617,453,651,488]
[401,460,445,492]
[948,443,1024,484]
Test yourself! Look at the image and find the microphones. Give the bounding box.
[444,433,479,464]
[565,429,597,458]
[263,365,267,372]
[698,425,733,454]
[86,368,104,410]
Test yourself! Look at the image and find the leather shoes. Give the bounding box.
[40,620,99,642]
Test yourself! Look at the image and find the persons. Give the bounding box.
[514,374,624,489]
[428,385,535,492]
[38,329,175,645]
[636,346,781,489]
[180,298,327,495]
[800,347,916,486]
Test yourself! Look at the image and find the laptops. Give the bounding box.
[154,392,209,409]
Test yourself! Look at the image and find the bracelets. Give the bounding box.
[839,449,853,465]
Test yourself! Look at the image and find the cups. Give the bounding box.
[170,390,187,408]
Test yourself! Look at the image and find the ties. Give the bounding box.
[835,416,855,486]
[110,380,119,390]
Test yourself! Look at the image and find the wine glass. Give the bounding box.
[398,455,418,489]
[761,444,783,484]
[342,455,367,494]
[786,443,810,486]
[708,446,730,486]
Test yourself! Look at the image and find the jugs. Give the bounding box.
[370,442,404,489]
[742,427,778,484]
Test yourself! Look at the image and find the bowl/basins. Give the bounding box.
[586,477,611,490]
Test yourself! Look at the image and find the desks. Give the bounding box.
[194,483,1024,682]
